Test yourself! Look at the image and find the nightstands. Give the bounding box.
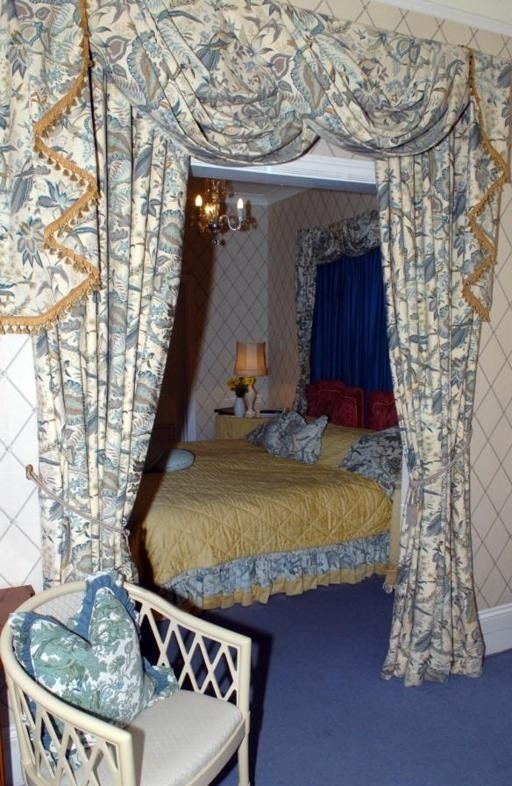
[213,413,276,440]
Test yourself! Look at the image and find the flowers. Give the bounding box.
[227,376,255,395]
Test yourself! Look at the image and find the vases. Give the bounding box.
[234,393,245,418]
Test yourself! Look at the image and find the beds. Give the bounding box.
[128,378,408,614]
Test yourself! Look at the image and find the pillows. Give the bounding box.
[166,448,195,474]
[10,571,177,775]
[338,425,402,502]
[246,406,330,465]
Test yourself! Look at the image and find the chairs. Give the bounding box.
[1,570,256,785]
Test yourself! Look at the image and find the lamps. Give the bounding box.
[234,341,269,417]
[182,172,258,247]
